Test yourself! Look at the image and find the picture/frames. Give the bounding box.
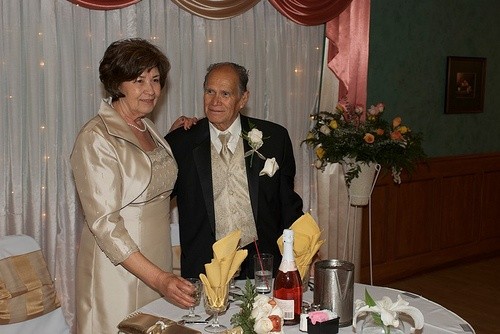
[444,56,488,114]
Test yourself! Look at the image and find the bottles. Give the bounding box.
[310,303,321,313]
[272,229,303,326]
[299,302,310,332]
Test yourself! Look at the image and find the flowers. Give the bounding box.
[238,119,271,168]
[300,103,427,185]
[220,276,284,334]
[351,286,423,334]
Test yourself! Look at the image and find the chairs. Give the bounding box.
[0,234,71,334]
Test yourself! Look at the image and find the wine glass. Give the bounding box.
[229,265,241,292]
[181,278,203,321]
[204,282,230,333]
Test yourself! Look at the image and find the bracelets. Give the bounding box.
[152,270,164,289]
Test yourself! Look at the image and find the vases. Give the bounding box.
[339,159,377,205]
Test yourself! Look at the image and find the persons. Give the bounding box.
[70,37,198,334]
[163,62,303,281]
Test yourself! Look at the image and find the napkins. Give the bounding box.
[276,212,326,279]
[200,230,249,311]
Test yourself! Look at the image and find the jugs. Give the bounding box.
[314,258,355,328]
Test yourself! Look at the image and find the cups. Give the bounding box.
[297,264,312,294]
[253,253,274,294]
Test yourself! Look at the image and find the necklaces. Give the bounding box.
[110,101,147,133]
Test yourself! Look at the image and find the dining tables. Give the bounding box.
[116,281,476,334]
